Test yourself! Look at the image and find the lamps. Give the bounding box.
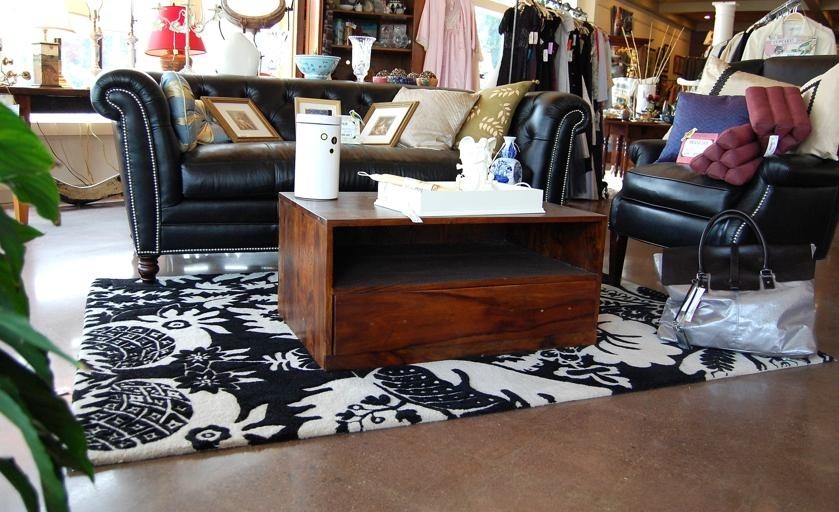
[145,7,206,74]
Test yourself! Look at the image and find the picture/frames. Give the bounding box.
[201,97,282,144]
[353,101,418,146]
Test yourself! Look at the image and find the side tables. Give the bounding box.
[602,119,671,188]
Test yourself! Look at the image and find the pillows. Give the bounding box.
[454,81,544,153]
[656,93,749,161]
[389,85,478,152]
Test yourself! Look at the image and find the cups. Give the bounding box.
[333,19,344,46]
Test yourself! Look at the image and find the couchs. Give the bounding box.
[91,70,593,285]
[610,139,839,286]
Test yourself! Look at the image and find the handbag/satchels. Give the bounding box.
[657,208,820,359]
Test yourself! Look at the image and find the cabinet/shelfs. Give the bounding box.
[306,1,418,81]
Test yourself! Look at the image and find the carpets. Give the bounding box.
[70,269,831,469]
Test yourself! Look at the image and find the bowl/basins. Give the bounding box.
[340,5,353,11]
[294,54,341,80]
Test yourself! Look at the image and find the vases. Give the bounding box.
[636,84,658,117]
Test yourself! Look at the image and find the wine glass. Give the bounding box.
[348,36,377,84]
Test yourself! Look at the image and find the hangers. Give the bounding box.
[508,0,588,85]
[726,2,801,32]
[518,0,585,17]
